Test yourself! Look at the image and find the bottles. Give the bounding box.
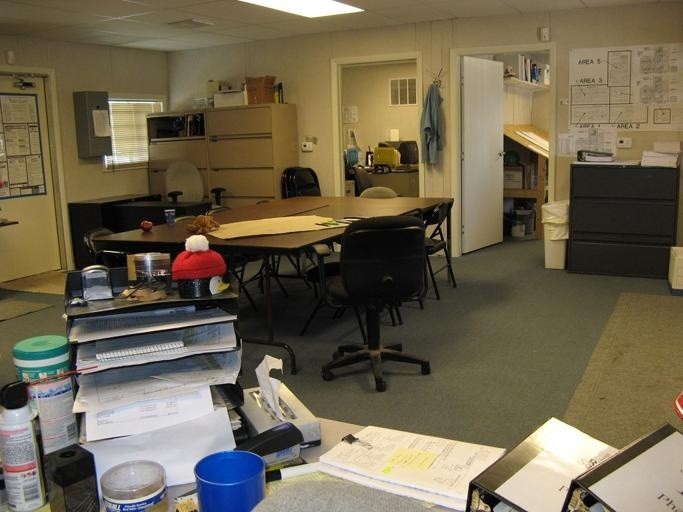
[133,253,172,291]
[366,146,373,166]
[99,457,171,512]
[1,332,97,511]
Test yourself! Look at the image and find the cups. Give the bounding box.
[193,449,268,511]
[163,209,176,223]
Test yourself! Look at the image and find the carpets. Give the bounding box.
[559,294,681,450]
[2,271,68,296]
[0,294,56,321]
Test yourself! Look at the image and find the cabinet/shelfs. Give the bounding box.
[565,164,679,280]
[501,123,549,241]
[203,102,298,210]
[145,108,209,201]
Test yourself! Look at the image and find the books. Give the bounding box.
[577,150,614,162]
[183,114,203,137]
[641,150,680,169]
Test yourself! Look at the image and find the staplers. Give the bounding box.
[233,422,308,471]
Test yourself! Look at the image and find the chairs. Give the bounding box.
[74,228,136,268]
[358,187,398,199]
[281,168,321,200]
[322,216,430,391]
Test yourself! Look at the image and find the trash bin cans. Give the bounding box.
[541,200,570,270]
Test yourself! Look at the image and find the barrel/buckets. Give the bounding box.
[510,208,536,233]
[503,212,526,237]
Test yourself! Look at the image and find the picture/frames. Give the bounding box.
[542,63,550,85]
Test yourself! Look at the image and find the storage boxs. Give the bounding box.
[212,89,248,107]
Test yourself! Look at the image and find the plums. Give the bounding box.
[140,220,152,231]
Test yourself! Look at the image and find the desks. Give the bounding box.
[93,194,455,375]
[2,397,506,512]
